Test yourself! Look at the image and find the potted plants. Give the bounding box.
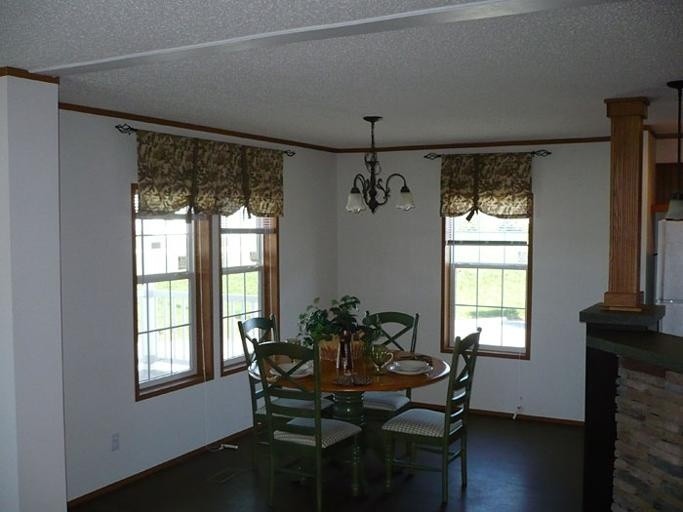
[296,294,365,361]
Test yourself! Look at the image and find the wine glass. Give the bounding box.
[371,351,387,376]
[284,337,300,363]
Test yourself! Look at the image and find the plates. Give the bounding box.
[389,360,434,376]
[269,364,312,378]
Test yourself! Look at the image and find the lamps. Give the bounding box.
[343,115,415,214]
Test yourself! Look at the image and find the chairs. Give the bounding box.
[382,327,481,508]
[237,314,334,468]
[252,339,364,511]
[362,312,420,461]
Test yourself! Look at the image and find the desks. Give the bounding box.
[247,344,449,468]
